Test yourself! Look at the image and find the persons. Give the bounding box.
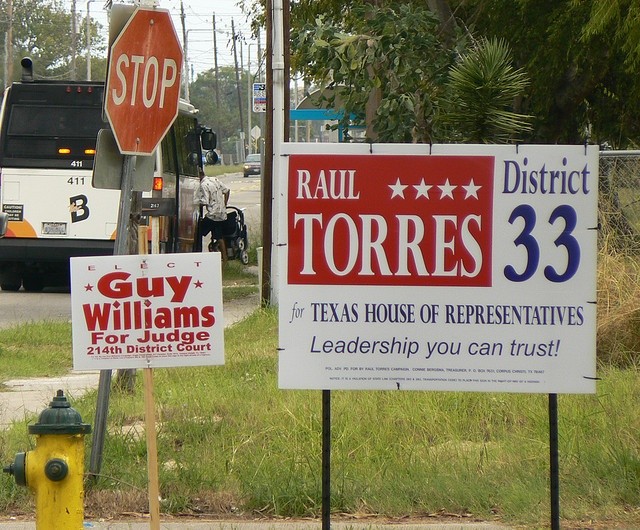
[199,171,231,265]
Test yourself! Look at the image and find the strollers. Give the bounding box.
[208,205,250,266]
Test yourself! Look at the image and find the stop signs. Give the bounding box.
[104,5,183,157]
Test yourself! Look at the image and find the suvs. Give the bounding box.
[241,153,261,177]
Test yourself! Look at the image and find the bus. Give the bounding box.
[0,78,218,293]
